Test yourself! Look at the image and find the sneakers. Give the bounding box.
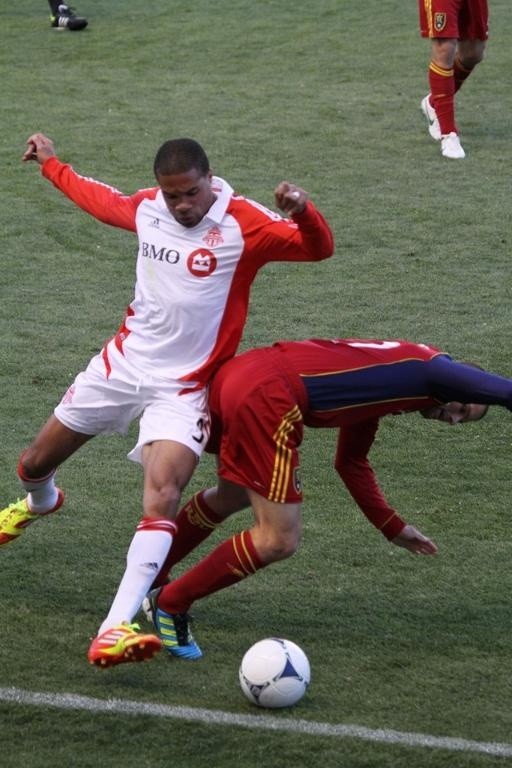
[49,4,88,32]
[0,485,66,545]
[86,625,163,670]
[441,131,467,159]
[419,92,444,141]
[140,584,204,661]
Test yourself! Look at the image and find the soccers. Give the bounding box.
[235,637,312,709]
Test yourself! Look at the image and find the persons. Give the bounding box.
[416,1,489,160]
[45,1,90,32]
[0,132,334,670]
[141,340,512,660]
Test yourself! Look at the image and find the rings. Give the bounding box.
[293,191,300,199]
